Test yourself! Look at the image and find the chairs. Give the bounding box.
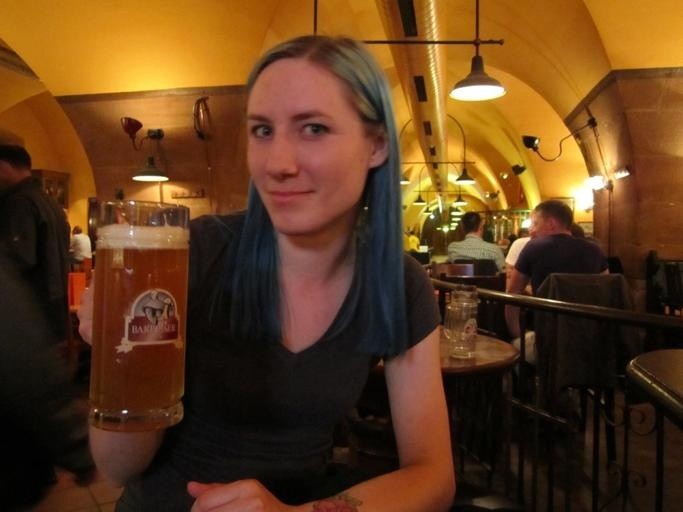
[428,255,682,511]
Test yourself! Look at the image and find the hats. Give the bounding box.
[0,129,25,146]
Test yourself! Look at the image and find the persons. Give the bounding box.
[0,144,71,343]
[77,35,456,511]
[70,225,92,263]
[409,230,419,253]
[448,200,609,367]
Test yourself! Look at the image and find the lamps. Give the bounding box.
[399,118,475,231]
[574,165,631,212]
[449,2,507,103]
[131,155,171,204]
[120,117,164,151]
[522,127,586,162]
[482,163,526,200]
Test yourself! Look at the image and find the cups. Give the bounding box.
[89,198,192,432]
[442,290,478,360]
